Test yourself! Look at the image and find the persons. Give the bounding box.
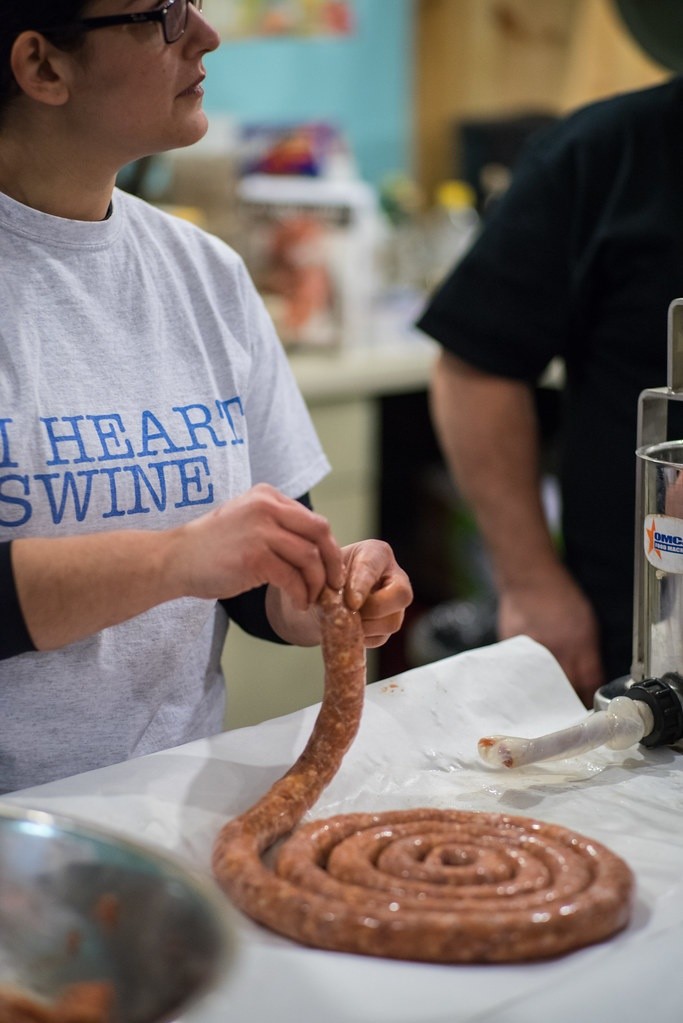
[1,4,414,798]
[418,2,683,709]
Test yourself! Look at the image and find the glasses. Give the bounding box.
[68,0,204,45]
